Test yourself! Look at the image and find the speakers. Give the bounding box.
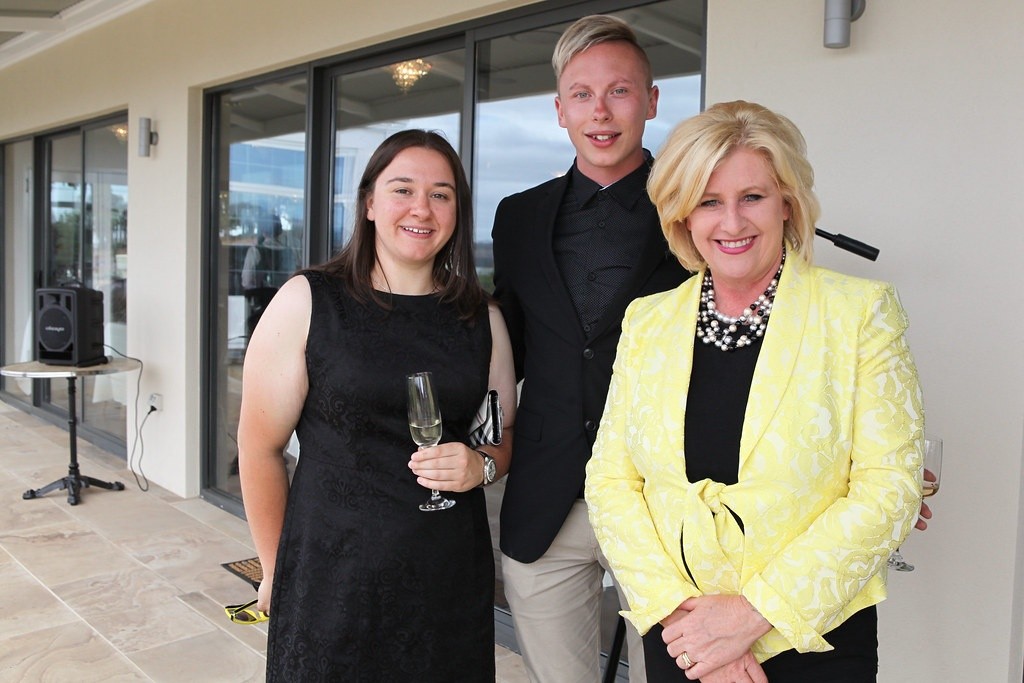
[33,279,108,369]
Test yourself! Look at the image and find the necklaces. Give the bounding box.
[693,254,786,350]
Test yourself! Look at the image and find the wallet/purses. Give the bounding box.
[469,389,505,448]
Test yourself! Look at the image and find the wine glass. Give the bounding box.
[884,435,942,573]
[405,370,456,511]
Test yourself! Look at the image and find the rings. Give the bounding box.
[682,652,694,668]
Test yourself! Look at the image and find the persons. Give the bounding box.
[233,131,517,682]
[241,215,294,366]
[484,11,938,680]
[582,102,924,680]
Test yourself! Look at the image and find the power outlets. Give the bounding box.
[149,393,164,413]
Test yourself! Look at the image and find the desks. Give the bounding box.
[0,359,142,507]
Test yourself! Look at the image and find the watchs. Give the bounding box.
[477,448,499,488]
